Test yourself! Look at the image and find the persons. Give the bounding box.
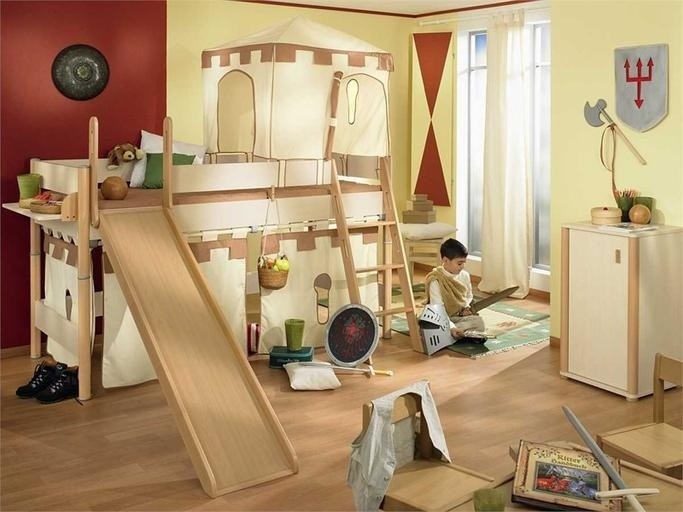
[421,239,487,344]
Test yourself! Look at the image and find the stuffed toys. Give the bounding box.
[106,142,144,169]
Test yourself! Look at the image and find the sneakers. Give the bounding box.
[15,361,79,403]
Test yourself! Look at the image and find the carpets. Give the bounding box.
[378,282,550,360]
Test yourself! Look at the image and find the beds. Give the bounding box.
[1,112,423,498]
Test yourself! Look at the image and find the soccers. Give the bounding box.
[628,204,652,225]
[101,175,129,201]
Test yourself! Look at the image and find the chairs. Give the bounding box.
[362,378,494,512]
[592,353,683,480]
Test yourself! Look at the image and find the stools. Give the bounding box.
[404,238,443,286]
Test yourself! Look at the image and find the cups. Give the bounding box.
[284,319,305,351]
[472,488,504,511]
[17,173,40,199]
[618,190,634,222]
[635,197,654,224]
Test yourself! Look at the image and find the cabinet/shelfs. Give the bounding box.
[558,220,681,403]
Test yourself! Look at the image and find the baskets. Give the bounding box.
[258,267,289,289]
[19,198,62,214]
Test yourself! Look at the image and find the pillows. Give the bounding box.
[396,222,458,241]
[127,129,208,190]
[280,361,342,391]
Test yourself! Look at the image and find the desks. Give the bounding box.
[435,441,682,511]
